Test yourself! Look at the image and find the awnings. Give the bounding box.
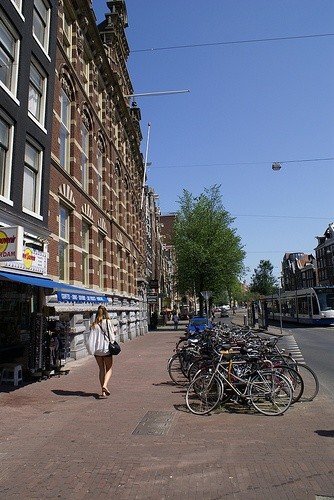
[0,272,109,303]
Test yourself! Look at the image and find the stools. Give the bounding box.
[0,365,23,386]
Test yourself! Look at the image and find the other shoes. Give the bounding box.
[102,387,111,396]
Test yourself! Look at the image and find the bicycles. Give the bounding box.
[167,315,320,417]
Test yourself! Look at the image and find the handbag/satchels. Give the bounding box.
[109,340,121,355]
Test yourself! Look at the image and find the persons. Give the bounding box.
[172,312,179,330]
[87,305,116,398]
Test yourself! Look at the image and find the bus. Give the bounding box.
[264,284,334,326]
